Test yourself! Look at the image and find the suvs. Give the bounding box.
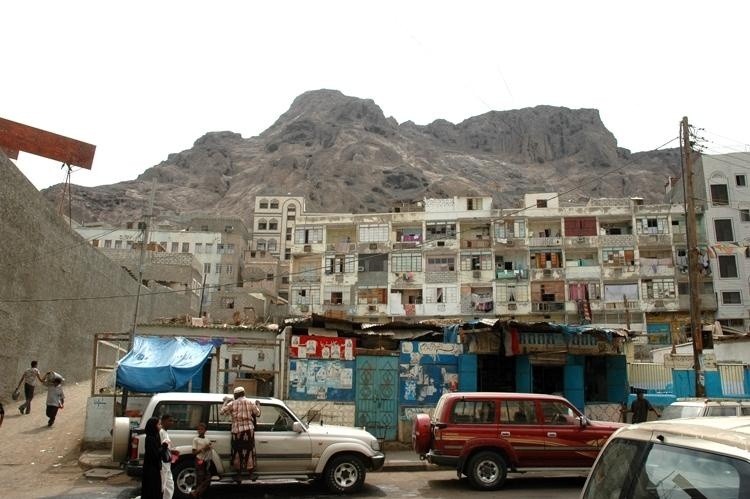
[578,419,750,499]
[112,392,384,494]
[412,392,631,489]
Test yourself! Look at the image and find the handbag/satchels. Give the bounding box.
[196,460,204,465]
[163,448,172,462]
[12,388,20,400]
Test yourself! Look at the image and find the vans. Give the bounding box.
[661,401,749,420]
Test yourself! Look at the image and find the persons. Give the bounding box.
[12,360,43,416]
[158,414,178,499]
[220,386,262,483]
[41,371,66,427]
[140,416,167,499]
[619,389,662,424]
[190,423,217,499]
[0,403,5,427]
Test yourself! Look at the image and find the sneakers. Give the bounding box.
[48,419,54,426]
[19,405,24,413]
[233,474,242,483]
[249,475,258,479]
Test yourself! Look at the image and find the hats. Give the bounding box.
[234,387,244,393]
